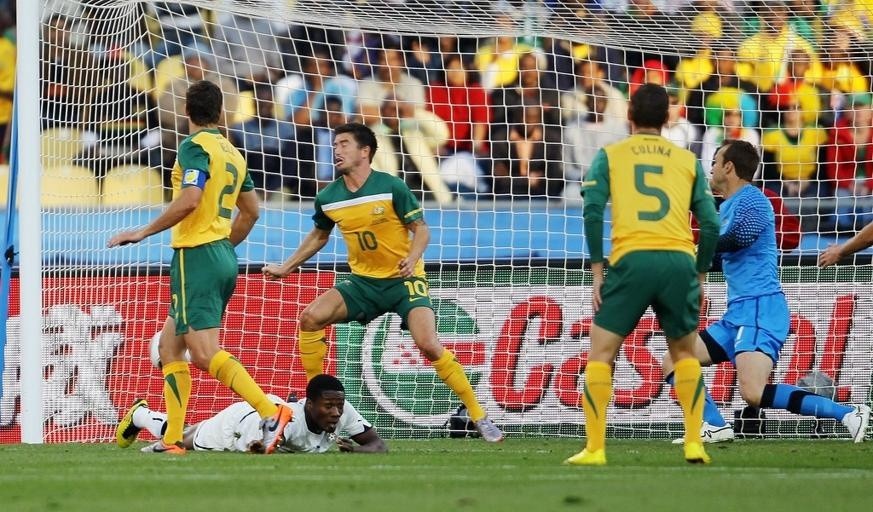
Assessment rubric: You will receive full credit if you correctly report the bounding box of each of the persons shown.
[106,80,293,455]
[818,221,873,270]
[566,84,721,464]
[691,147,800,272]
[0,0,873,225]
[670,140,870,444]
[261,124,504,442]
[116,374,386,454]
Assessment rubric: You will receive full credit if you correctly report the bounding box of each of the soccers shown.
[149,328,194,370]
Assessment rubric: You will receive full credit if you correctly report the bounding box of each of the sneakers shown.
[841,403,872,444]
[684,444,710,464]
[116,398,148,448]
[671,422,734,445]
[139,440,187,454]
[260,402,294,453]
[567,449,606,465]
[472,413,503,443]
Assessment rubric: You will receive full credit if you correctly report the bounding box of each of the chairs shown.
[0,125,164,209]
